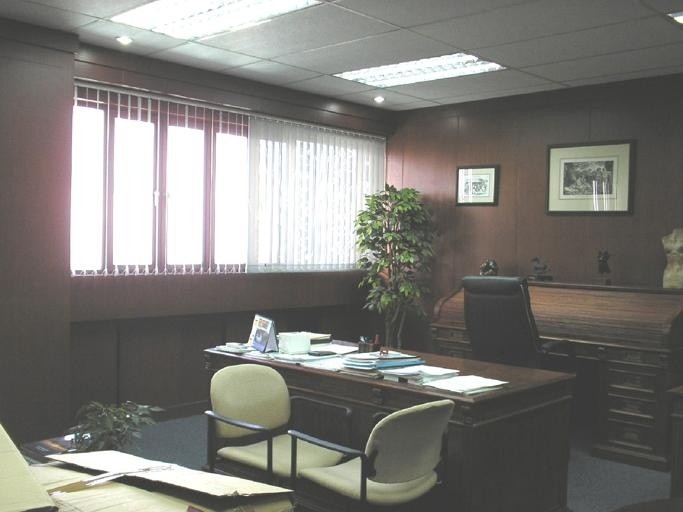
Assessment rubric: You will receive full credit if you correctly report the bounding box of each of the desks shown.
[203,330,576,512]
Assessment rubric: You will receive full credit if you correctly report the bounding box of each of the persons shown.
[660,227,682,288]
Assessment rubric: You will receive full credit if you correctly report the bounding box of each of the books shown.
[343,346,510,398]
[215,330,361,373]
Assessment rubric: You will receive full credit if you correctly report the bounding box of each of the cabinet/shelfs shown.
[428,281,681,474]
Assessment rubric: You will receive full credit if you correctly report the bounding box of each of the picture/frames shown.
[544,139,633,214]
[454,164,498,206]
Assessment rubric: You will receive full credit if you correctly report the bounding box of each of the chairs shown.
[201,364,454,512]
[461,274,542,369]
